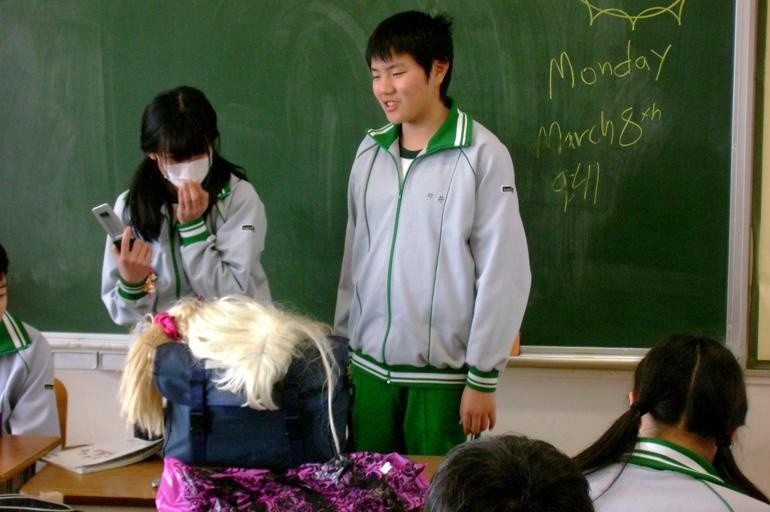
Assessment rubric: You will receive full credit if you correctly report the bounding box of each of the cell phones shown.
[92,203,135,254]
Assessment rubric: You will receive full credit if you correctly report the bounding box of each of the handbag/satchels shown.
[159,336,352,468]
[156,451,428,511]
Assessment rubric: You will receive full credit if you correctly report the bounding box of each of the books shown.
[38,434,166,476]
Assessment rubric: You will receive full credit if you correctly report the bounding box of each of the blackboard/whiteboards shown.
[0,0,760,371]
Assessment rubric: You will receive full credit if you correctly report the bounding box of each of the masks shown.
[153,145,213,189]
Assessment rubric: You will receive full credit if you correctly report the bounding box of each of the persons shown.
[421,432,595,512]
[570,333,769,512]
[333,8,532,454]
[100,86,275,327]
[0,247,67,476]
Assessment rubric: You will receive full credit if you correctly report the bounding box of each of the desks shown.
[20,445,446,511]
[0,433,64,494]
